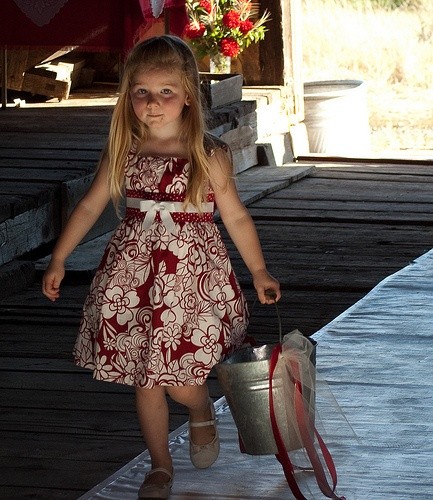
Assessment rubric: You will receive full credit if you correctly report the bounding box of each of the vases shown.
[209,56,231,73]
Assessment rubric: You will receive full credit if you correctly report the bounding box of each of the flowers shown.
[184,0,273,70]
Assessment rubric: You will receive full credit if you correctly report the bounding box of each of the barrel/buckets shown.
[215,292,318,456]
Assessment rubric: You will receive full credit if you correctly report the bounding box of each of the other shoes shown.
[138,465,175,498]
[188,397,219,470]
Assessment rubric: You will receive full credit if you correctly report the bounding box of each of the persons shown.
[43,34,282,499]
